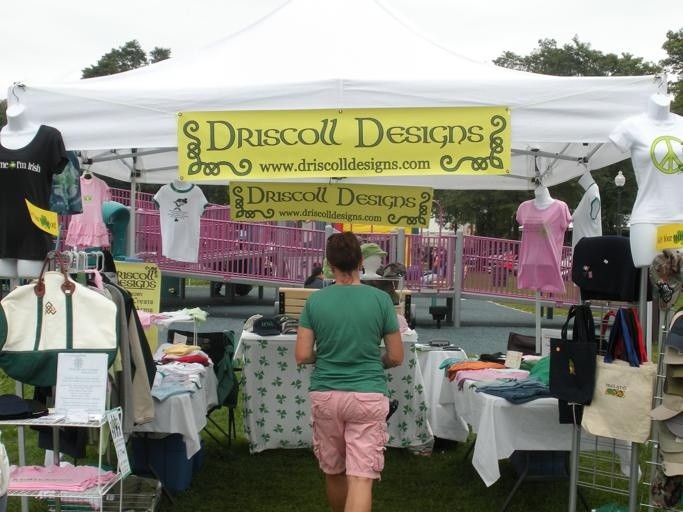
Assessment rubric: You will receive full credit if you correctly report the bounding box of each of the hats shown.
[648,250,683,508]
[359,242,388,260]
[244,314,281,335]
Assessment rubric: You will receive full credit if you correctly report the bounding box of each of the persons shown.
[571,172,602,260]
[0,104,68,280]
[609,96,683,266]
[295,232,404,512]
[493,247,512,288]
[427,244,447,276]
[516,188,564,267]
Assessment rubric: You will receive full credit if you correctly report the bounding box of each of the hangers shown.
[75,160,109,193]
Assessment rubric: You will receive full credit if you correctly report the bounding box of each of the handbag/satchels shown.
[0,250,120,387]
[548,304,658,443]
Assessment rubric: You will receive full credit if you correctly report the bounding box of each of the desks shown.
[234,316,423,458]
[0,413,133,512]
[440,351,592,512]
[133,342,221,505]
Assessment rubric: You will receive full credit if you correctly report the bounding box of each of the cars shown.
[486,252,519,275]
